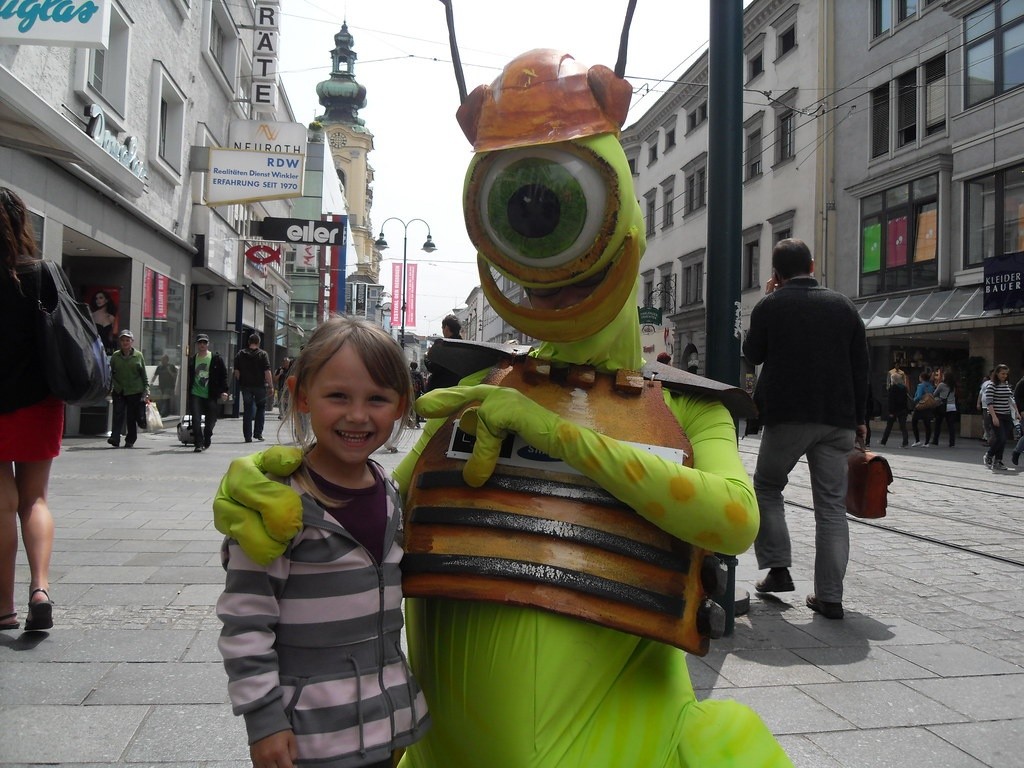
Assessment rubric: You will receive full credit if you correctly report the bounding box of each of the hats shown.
[196,334,210,343]
[120,329,134,338]
[657,353,671,364]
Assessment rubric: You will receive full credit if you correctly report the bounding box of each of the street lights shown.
[373,217,439,347]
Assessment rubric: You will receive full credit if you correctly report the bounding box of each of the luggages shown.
[177,414,206,445]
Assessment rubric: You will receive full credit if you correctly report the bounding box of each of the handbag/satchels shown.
[134,401,147,429]
[916,393,936,409]
[146,402,164,432]
[38,259,114,405]
[932,397,948,413]
[846,436,894,519]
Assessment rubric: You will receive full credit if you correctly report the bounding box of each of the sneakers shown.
[756,569,796,593]
[806,594,846,620]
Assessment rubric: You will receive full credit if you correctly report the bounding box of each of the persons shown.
[213,47,798,768]
[107,328,149,448]
[976,363,1024,470]
[187,333,229,454]
[149,354,179,417]
[384,361,427,453]
[214,314,433,768]
[0,184,77,632]
[233,333,274,443]
[423,315,465,391]
[274,357,291,421]
[742,236,869,620]
[865,361,957,449]
[656,352,672,365]
[89,290,119,355]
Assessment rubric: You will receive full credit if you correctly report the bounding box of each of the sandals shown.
[0,588,54,630]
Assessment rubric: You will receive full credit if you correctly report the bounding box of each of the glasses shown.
[198,341,207,344]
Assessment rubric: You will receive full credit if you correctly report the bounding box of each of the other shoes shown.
[107,437,133,448]
[984,454,1008,470]
[877,440,886,447]
[391,446,399,453]
[911,441,930,448]
[194,442,211,452]
[899,445,909,448]
[245,435,265,443]
